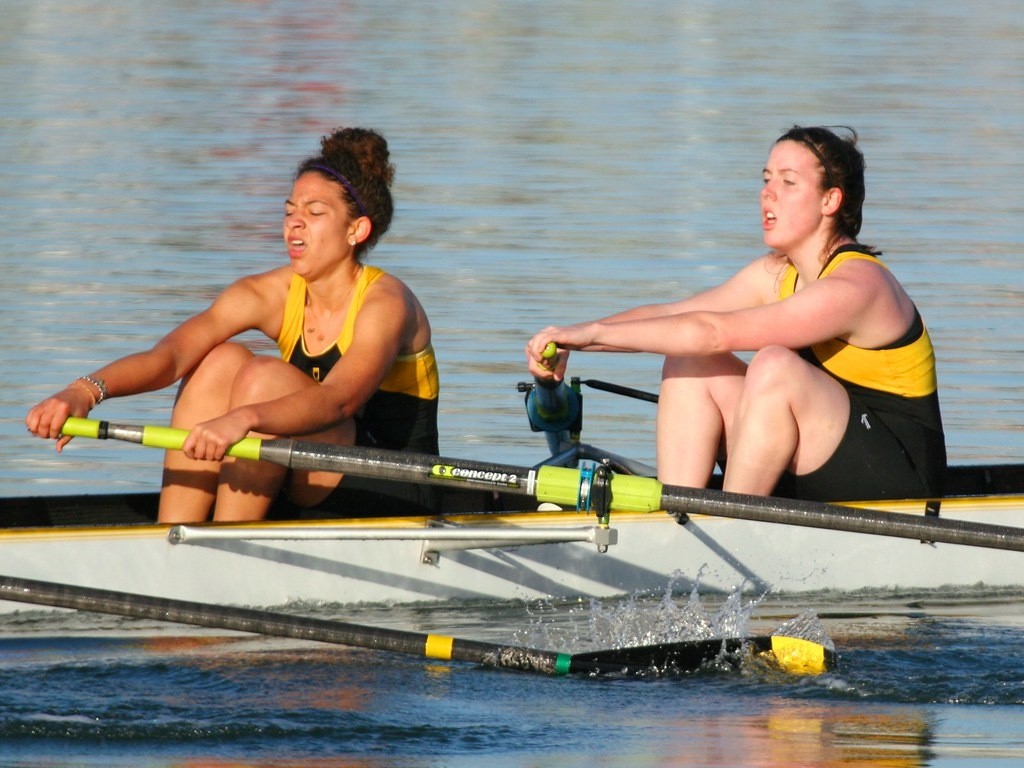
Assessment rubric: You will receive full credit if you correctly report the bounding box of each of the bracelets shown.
[72,374,106,409]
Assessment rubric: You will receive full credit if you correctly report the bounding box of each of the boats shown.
[0,461,1024,617]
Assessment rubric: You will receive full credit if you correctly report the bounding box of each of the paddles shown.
[1,573,843,689]
[57,413,1024,554]
[517,340,586,468]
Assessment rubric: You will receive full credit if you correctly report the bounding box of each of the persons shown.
[526,123,949,510]
[19,127,451,530]
